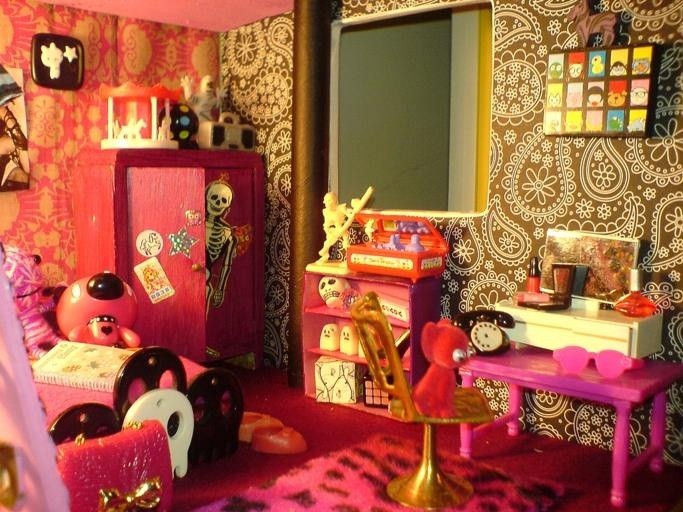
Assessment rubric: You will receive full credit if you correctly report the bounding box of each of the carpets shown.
[195,433,568,511]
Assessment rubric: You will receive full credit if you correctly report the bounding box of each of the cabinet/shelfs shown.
[73,149,264,374]
[301,269,441,424]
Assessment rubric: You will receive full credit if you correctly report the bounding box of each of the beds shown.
[29,345,246,480]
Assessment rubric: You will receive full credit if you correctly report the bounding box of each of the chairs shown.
[350,292,495,512]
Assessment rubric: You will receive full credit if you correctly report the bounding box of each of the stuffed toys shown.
[0,241,60,359]
[403,315,470,418]
